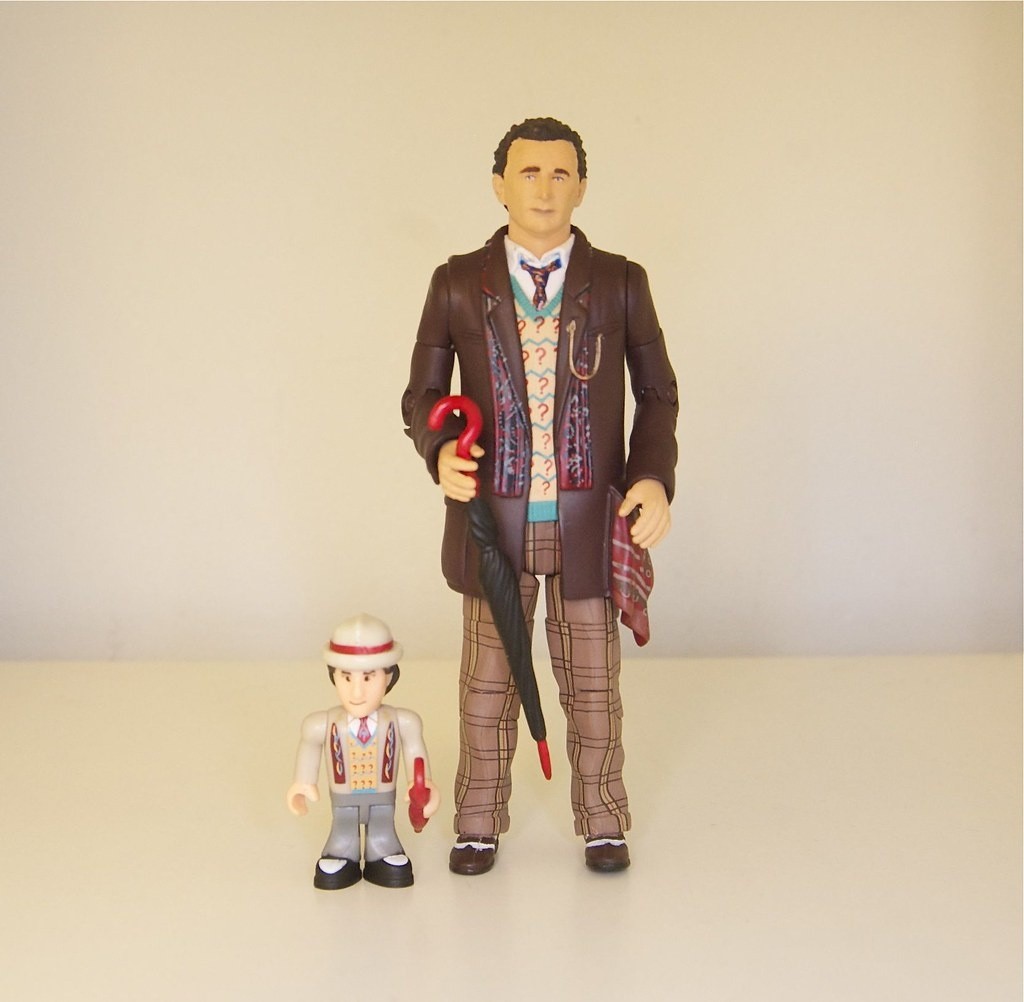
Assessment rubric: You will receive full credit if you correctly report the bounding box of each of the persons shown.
[288,609,441,890]
[401,113,680,872]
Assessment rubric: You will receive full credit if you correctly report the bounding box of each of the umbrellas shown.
[427,396,551,780]
[408,758,431,833]
